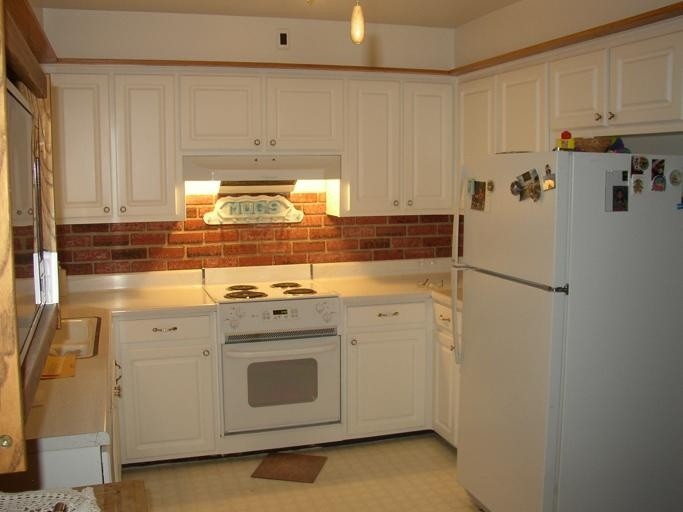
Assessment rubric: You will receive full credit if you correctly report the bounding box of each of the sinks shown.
[46,317,101,358]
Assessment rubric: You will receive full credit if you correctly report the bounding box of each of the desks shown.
[0,480,151,512]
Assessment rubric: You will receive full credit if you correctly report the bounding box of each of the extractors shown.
[180,153,342,182]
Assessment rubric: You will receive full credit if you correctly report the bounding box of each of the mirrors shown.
[6,82,42,367]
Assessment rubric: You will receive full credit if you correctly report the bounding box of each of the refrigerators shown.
[449,153,683,512]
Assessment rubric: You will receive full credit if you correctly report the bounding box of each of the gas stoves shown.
[201,280,336,303]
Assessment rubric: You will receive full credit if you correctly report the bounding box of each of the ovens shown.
[222,336,340,434]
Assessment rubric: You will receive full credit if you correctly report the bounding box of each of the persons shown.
[614,188,626,211]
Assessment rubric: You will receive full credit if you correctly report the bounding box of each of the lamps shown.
[350,1,364,45]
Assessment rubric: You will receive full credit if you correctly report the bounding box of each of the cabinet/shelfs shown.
[325,77,460,217]
[38,362,123,487]
[182,71,345,180]
[434,304,460,443]
[558,18,683,134]
[460,64,546,156]
[48,68,186,223]
[112,311,215,470]
[343,300,435,446]
